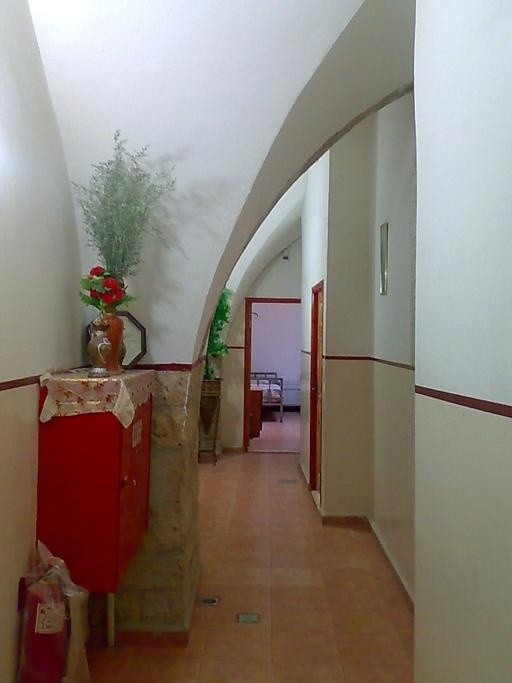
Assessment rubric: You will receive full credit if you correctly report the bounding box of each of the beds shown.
[250,371,283,423]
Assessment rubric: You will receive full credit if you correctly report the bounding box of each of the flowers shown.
[79,268,132,308]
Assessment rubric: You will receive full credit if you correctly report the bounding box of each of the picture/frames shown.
[85,310,147,370]
[379,221,389,295]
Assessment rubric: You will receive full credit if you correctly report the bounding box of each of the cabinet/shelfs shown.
[249,386,263,440]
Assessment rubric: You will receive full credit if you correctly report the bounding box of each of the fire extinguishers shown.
[15,566,70,683]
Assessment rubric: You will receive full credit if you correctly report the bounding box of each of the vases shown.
[103,313,125,373]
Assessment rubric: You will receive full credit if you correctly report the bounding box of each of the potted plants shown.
[200,286,234,436]
